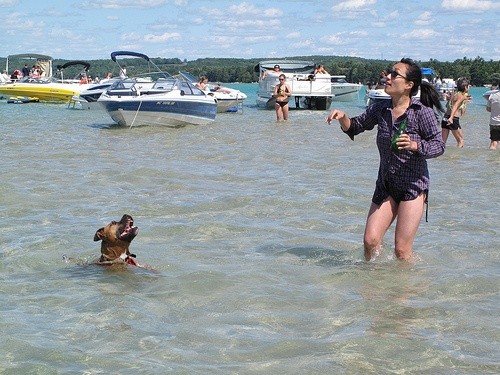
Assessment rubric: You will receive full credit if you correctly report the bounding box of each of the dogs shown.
[87,214,160,275]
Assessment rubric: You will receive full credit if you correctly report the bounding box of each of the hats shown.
[457,78,470,87]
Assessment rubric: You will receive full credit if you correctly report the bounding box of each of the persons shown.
[0,63,129,85]
[486,81,500,149]
[367,69,459,101]
[195,76,209,91]
[270,64,327,74]
[441,78,470,147]
[274,74,292,122]
[326,58,447,263]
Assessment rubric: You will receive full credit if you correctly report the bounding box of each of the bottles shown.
[389,117,408,154]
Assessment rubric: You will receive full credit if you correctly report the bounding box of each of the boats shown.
[158,71,248,113]
[367,67,498,105]
[0,53,99,104]
[253,58,335,110]
[77,75,159,110]
[330,74,368,103]
[99,50,219,129]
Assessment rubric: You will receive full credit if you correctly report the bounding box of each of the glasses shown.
[279,78,284,81]
[386,68,412,82]
